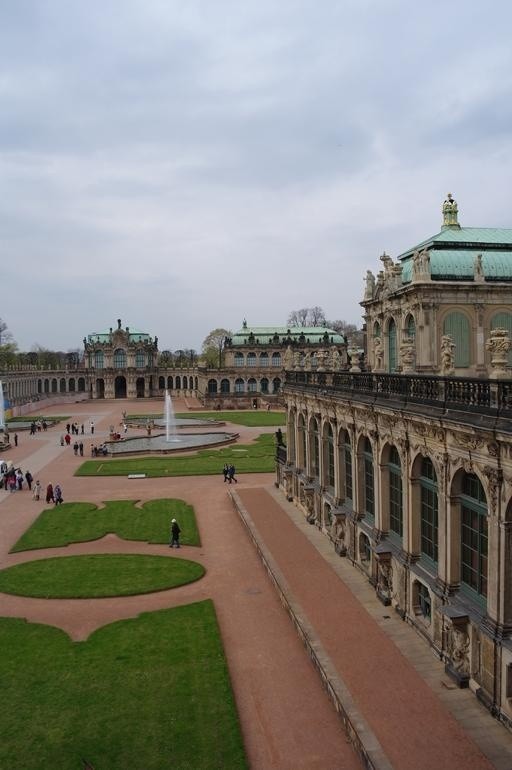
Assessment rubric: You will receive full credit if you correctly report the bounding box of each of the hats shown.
[171,519,176,522]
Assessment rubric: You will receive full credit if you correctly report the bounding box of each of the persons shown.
[3,464,63,506]
[168,518,181,548]
[223,463,238,483]
[29,419,48,435]
[146,423,152,435]
[14,433,18,446]
[124,423,127,433]
[59,422,121,457]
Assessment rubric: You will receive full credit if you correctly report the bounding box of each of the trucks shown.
[0,460,20,488]
